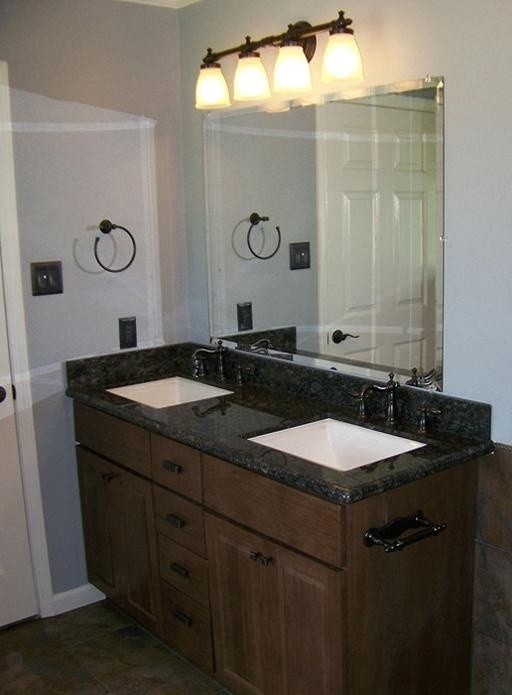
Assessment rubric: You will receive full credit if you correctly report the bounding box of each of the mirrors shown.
[202,76,445,390]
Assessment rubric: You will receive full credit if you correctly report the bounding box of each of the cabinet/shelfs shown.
[74,398,480,695]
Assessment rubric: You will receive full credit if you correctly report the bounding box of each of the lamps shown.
[195,11,367,110]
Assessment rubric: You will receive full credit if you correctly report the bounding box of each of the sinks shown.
[234,409,429,475]
[104,375,235,410]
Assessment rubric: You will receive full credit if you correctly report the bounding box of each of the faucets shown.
[406,365,439,388]
[357,372,403,426]
[189,396,232,417]
[235,334,273,356]
[190,339,229,383]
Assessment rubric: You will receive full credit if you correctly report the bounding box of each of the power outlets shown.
[118,316,137,349]
[236,303,254,332]
